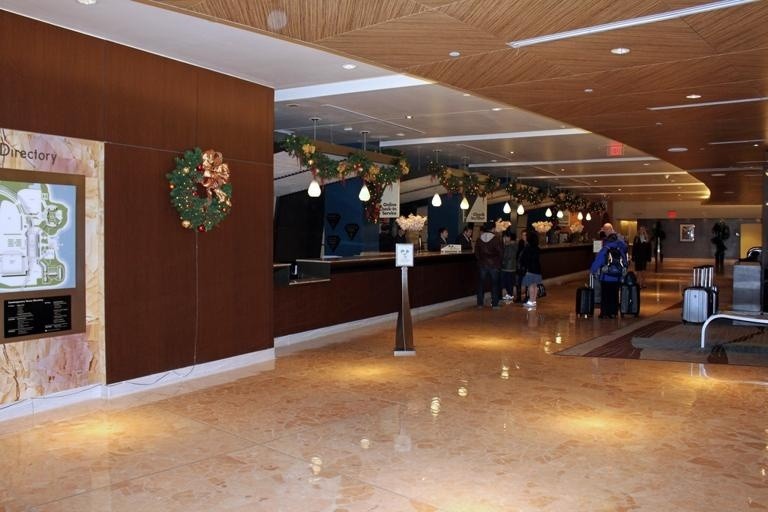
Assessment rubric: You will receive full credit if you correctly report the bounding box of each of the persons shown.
[378,219,653,319]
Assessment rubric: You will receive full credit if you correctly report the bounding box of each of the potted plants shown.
[710,216,731,273]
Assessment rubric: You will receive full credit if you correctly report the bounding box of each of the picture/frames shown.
[679,223,695,243]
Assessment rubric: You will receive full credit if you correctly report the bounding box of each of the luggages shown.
[577,273,639,318]
[682,265,719,325]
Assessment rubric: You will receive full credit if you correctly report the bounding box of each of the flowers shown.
[569,221,585,233]
[394,212,429,233]
[530,219,554,233]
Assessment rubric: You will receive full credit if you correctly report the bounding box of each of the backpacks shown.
[607,246,624,273]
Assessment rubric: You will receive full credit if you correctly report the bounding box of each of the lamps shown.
[307,114,322,199]
[358,130,372,204]
[430,148,592,222]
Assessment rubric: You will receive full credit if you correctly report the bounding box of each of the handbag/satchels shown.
[526,284,545,298]
[626,271,636,286]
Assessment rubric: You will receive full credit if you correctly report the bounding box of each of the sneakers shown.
[502,295,514,300]
[523,301,536,307]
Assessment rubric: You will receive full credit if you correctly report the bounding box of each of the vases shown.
[404,230,419,254]
[538,231,547,248]
